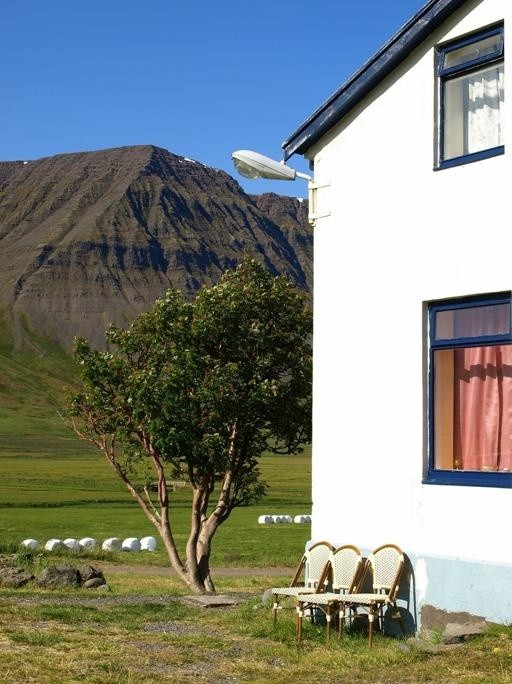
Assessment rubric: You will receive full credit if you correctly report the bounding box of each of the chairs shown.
[271,542,407,649]
